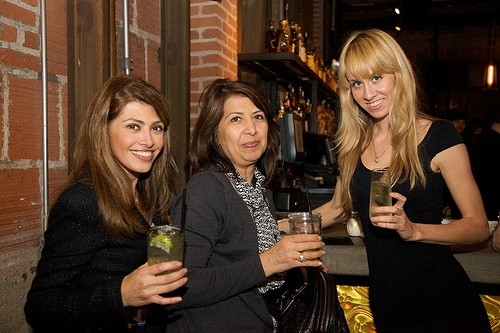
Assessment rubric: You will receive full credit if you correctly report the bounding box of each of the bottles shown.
[268,18,340,96]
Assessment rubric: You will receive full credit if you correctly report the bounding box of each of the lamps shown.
[479,0,500,90]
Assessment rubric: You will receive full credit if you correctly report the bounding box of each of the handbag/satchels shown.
[269,269,351,333]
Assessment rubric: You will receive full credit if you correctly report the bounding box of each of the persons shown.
[426,107,500,221]
[279,30,492,333]
[24,75,188,333]
[163,78,326,333]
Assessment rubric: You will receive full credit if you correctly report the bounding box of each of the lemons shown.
[149,235,173,254]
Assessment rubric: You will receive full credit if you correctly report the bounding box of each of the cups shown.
[146,226,183,276]
[369,168,393,226]
[288,211,323,263]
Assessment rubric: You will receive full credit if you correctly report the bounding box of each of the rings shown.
[297,252,306,262]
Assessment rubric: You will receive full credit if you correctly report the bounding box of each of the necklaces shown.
[134,191,139,204]
[371,137,391,163]
